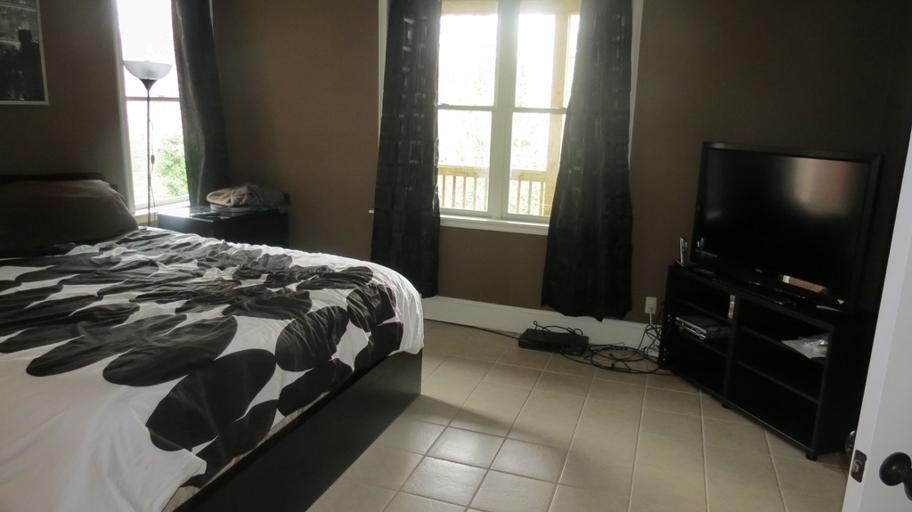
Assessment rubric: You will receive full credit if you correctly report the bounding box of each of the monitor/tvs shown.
[689,139,889,320]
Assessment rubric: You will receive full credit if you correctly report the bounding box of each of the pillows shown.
[0,180,137,257]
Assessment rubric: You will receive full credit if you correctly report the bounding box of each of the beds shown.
[0,171,424,512]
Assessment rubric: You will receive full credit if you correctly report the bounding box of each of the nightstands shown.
[158,206,288,247]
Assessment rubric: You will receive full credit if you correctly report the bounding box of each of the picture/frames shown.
[0,0,51,106]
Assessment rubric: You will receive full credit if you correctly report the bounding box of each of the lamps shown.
[122,60,173,226]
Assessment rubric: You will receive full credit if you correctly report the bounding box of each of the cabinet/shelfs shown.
[657,263,876,460]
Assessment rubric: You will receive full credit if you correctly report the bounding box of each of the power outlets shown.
[644,296,657,315]
